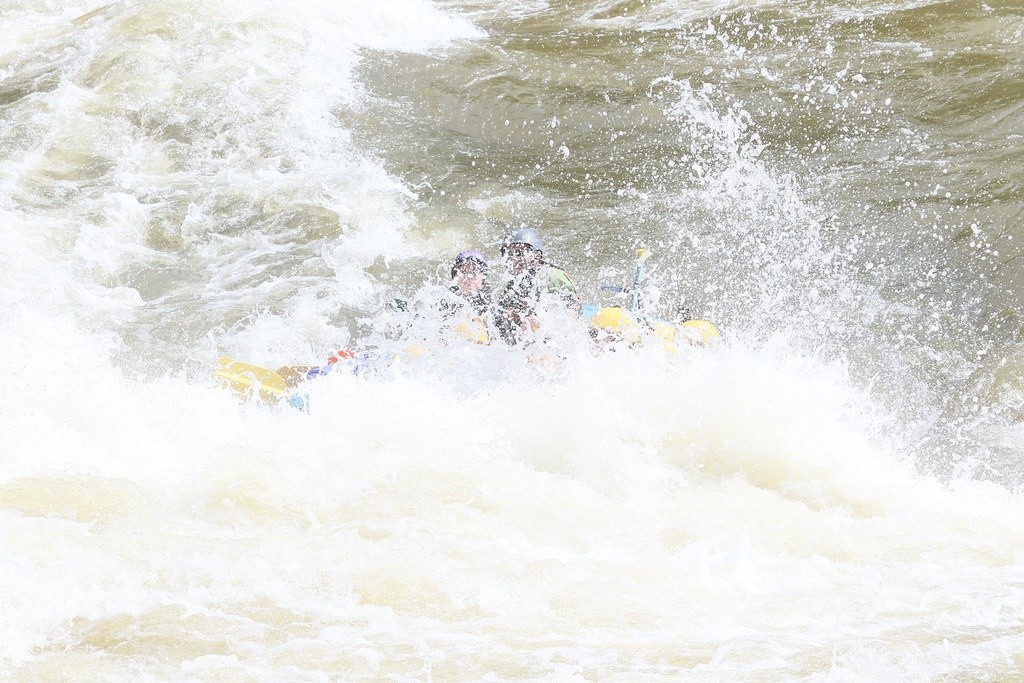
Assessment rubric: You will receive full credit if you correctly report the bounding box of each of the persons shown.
[492,228,583,348]
[446,252,515,345]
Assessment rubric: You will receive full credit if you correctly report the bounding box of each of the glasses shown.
[503,249,528,257]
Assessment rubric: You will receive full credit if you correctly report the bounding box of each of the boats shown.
[211,304,723,409]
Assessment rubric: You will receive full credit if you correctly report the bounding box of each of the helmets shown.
[451,250,488,279]
[500,227,543,258]
